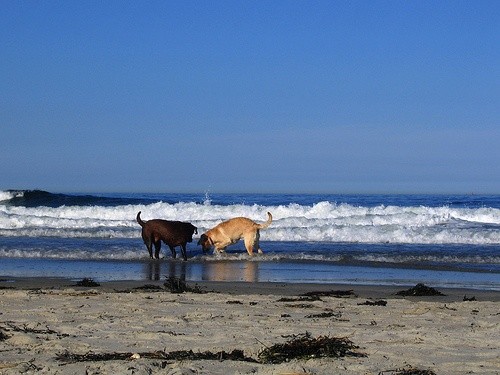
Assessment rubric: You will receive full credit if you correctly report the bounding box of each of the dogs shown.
[136,211,197,261]
[197,212,272,256]
